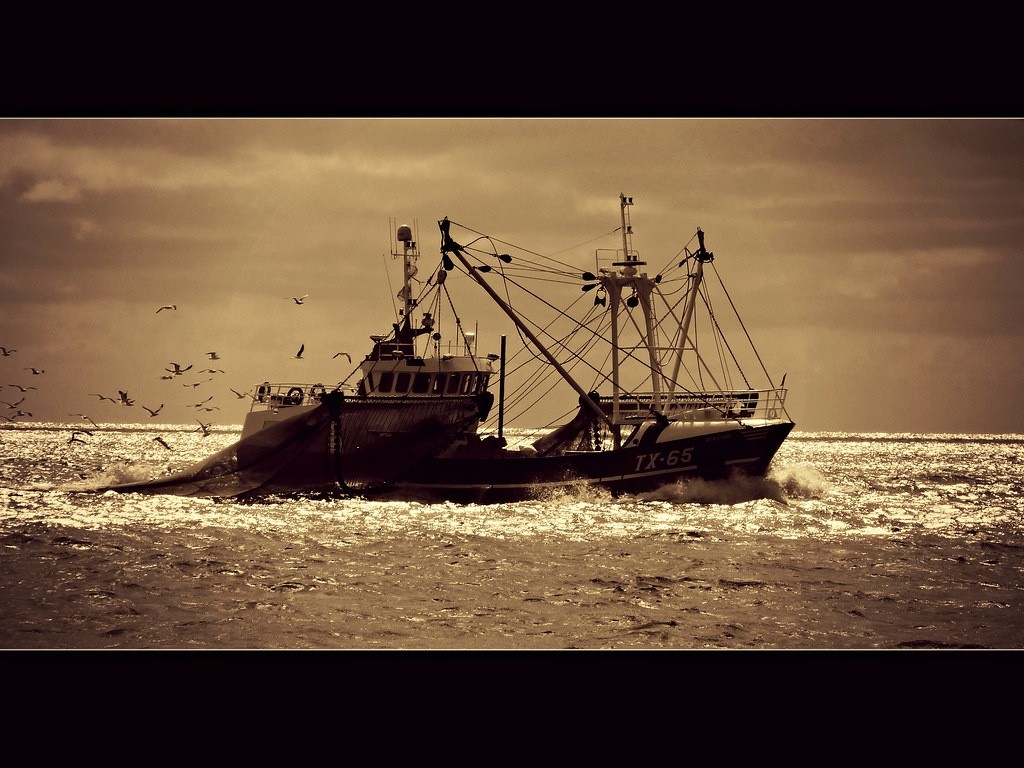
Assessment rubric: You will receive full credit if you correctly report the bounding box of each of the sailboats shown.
[405,192,797,500]
[69,216,495,503]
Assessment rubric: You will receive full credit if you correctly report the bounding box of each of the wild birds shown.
[155,303,177,314]
[332,352,352,364]
[290,344,306,361]
[230,388,247,399]
[284,294,311,306]
[0,344,226,451]
